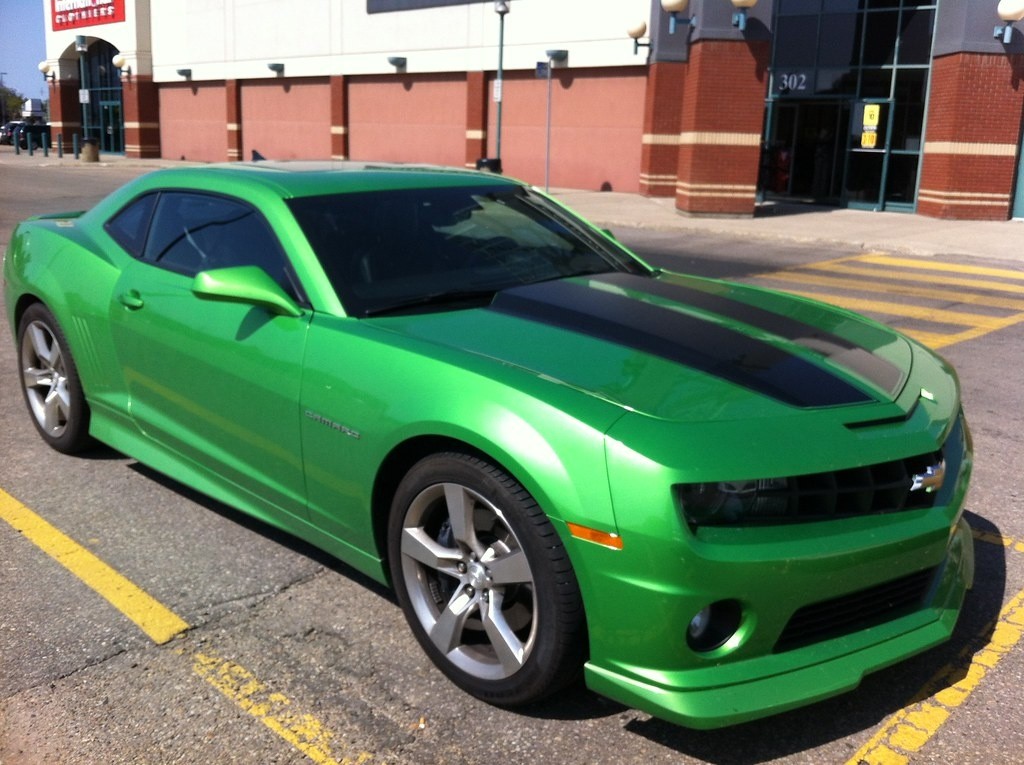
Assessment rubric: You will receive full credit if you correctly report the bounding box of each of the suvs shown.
[12,124,50,150]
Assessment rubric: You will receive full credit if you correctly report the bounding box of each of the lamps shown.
[994,0,1024,43]
[660,0,696,35]
[388,56,407,68]
[39,61,56,81]
[268,63,284,70]
[177,69,190,75]
[112,55,132,77]
[623,21,653,54]
[728,0,757,29]
[547,51,570,62]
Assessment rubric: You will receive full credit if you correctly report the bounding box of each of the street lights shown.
[74,34,101,163]
[495,3,512,170]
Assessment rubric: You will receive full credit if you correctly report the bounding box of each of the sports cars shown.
[11,160,984,739]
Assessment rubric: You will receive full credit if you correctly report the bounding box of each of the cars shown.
[0,121,19,144]
